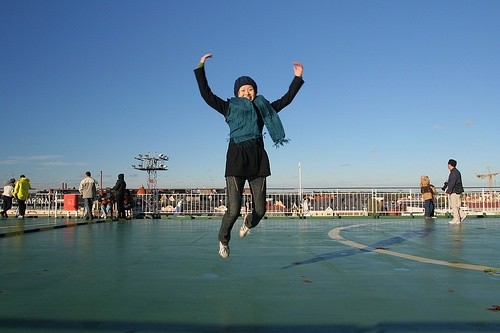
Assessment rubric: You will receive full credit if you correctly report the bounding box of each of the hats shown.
[234,76,258,97]
[449,159,457,164]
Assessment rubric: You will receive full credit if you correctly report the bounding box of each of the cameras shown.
[441,182,449,191]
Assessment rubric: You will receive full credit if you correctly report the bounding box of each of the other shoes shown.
[0,211,4,218]
[17,215,24,219]
[5,214,8,218]
[117,216,126,219]
[219,241,230,258]
[461,215,467,222]
[449,221,460,224]
[240,215,250,238]
[104,214,107,219]
[424,216,434,219]
[101,214,104,217]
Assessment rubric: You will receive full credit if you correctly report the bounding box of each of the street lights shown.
[131,152,169,218]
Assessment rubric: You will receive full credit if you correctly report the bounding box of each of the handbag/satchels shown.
[93,182,96,199]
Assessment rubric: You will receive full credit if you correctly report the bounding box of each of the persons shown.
[98,187,113,219]
[79,172,96,220]
[112,174,126,218]
[421,176,437,219]
[446,159,467,224]
[13,175,30,218]
[0,178,15,218]
[194,54,304,257]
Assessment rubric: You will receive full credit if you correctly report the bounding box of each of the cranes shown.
[474,166,500,194]
